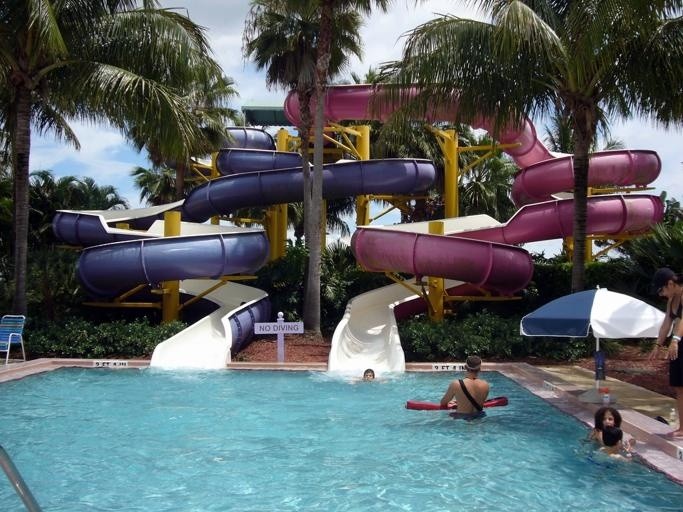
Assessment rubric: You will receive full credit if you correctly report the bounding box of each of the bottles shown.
[669,408,677,427]
[603,394,610,403]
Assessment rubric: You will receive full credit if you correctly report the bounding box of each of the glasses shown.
[655,283,666,295]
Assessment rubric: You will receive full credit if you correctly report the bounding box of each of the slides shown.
[52,85,663,372]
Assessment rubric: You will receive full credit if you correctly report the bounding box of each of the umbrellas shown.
[519,288,674,404]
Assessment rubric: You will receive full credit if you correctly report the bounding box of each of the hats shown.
[650,267,674,296]
[464,355,483,370]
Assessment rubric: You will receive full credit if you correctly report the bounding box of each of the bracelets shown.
[672,335,681,341]
[626,453,632,457]
[656,343,662,348]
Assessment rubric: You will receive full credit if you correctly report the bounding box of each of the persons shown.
[440,356,490,420]
[587,406,636,453]
[599,426,633,463]
[364,368,374,379]
[646,266,683,440]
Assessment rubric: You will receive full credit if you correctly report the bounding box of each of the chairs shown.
[0,314,27,364]
